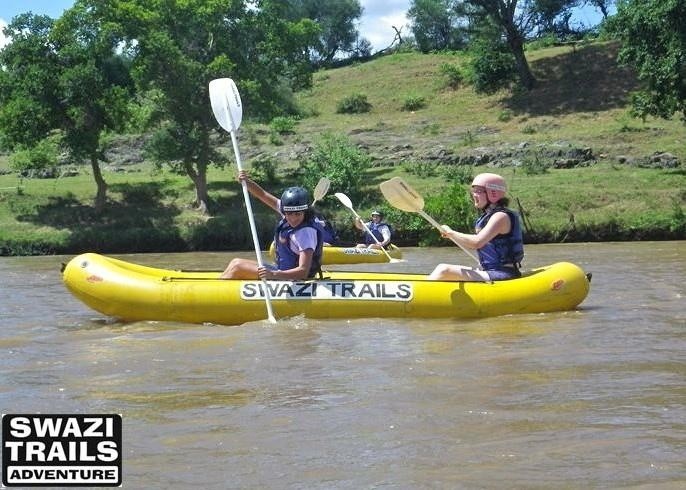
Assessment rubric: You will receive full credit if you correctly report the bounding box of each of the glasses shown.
[473,189,486,194]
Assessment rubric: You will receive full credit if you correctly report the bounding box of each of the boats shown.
[60,241,593,322]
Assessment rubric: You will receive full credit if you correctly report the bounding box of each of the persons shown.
[426,172,524,282]
[354,207,393,251]
[220,169,326,281]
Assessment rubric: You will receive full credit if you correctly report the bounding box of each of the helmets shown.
[471,172,506,203]
[277,186,309,215]
[371,210,381,217]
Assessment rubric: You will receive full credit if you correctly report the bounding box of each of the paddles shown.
[311,177,331,206]
[208,78,277,325]
[381,176,482,269]
[334,193,401,263]
